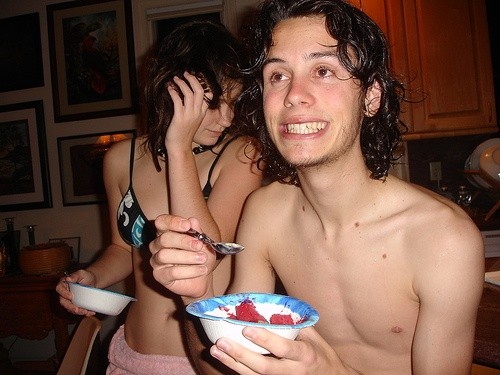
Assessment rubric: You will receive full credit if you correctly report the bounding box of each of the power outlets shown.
[430,161,442,182]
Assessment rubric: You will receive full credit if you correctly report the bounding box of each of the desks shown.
[0,262,89,375]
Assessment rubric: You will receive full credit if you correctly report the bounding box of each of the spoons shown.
[169,228,245,255]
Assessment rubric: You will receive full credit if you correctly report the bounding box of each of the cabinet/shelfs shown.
[344,0,498,140]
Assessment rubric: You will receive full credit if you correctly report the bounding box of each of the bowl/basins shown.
[463,138,500,189]
[19,243,66,276]
[68,279,134,316]
[185,292,320,354]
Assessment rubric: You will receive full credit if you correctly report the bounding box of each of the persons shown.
[55,20,267,375]
[147,2,487,375]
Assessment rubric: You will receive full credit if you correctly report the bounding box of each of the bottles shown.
[428,144,442,191]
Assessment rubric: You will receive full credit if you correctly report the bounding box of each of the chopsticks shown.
[62,271,80,284]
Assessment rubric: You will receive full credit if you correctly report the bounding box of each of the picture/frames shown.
[0,99,53,212]
[46,0,140,123]
[56,129,138,206]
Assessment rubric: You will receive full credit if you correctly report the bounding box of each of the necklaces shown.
[156,132,226,158]
